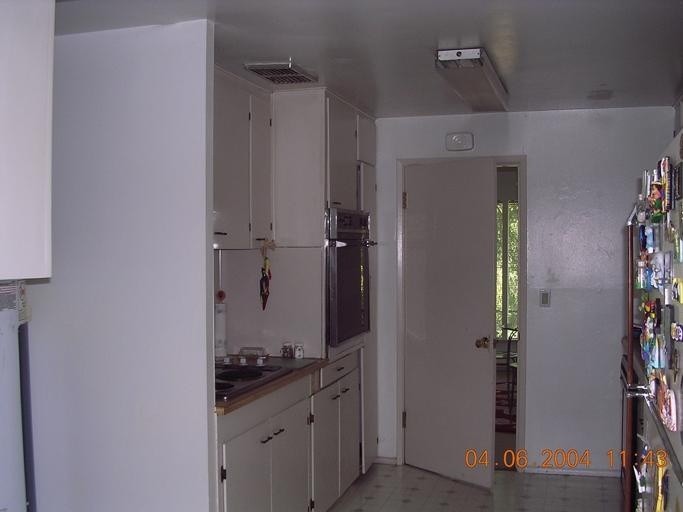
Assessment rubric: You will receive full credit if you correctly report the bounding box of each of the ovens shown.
[325,208,378,349]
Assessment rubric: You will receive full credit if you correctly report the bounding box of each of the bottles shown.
[294,343,305,360]
[282,343,293,359]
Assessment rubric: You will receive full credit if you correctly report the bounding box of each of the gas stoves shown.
[212,365,294,403]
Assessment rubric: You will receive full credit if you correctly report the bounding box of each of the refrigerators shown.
[620,205,647,510]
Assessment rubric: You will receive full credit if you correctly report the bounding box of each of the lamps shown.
[432,46,510,114]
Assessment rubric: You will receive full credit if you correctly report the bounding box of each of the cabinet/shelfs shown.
[214,64,272,249]
[273,87,377,247]
[312,342,377,512]
[218,398,310,511]
